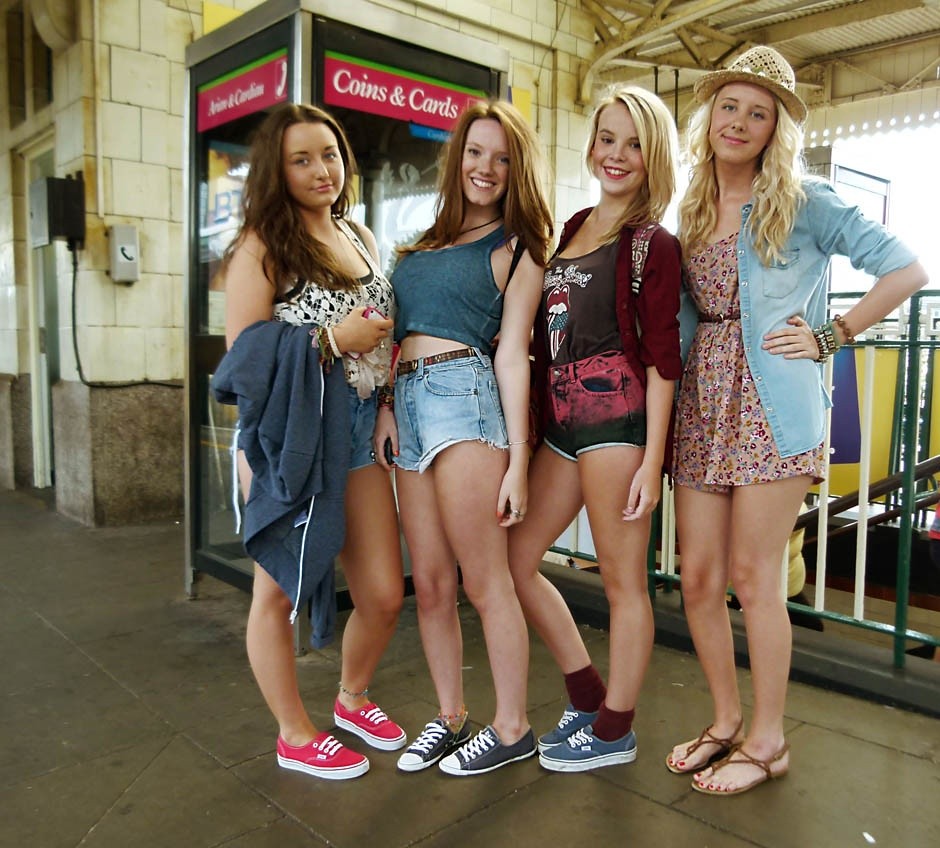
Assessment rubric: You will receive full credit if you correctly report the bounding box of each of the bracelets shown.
[309,324,344,374]
[812,321,841,363]
[378,394,393,407]
[508,440,530,445]
[835,315,855,347]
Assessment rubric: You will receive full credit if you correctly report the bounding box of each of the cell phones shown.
[345,306,389,360]
[386,438,392,465]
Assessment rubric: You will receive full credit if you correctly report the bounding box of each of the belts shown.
[398,347,485,376]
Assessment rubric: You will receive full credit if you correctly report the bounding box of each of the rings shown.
[518,512,525,519]
[511,510,519,518]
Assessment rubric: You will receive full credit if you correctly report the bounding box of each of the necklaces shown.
[457,215,502,234]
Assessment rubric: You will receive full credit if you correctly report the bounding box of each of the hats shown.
[693,44,807,125]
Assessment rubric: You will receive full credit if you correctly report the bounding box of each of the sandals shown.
[691,739,792,795]
[665,715,744,774]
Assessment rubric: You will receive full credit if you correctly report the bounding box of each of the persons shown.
[374,100,554,776]
[215,103,408,782]
[508,85,684,773]
[666,45,930,796]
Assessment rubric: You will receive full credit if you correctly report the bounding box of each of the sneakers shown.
[540,723,638,773]
[441,726,537,777]
[537,705,602,753]
[276,734,371,781]
[396,713,472,772]
[333,692,407,751]
